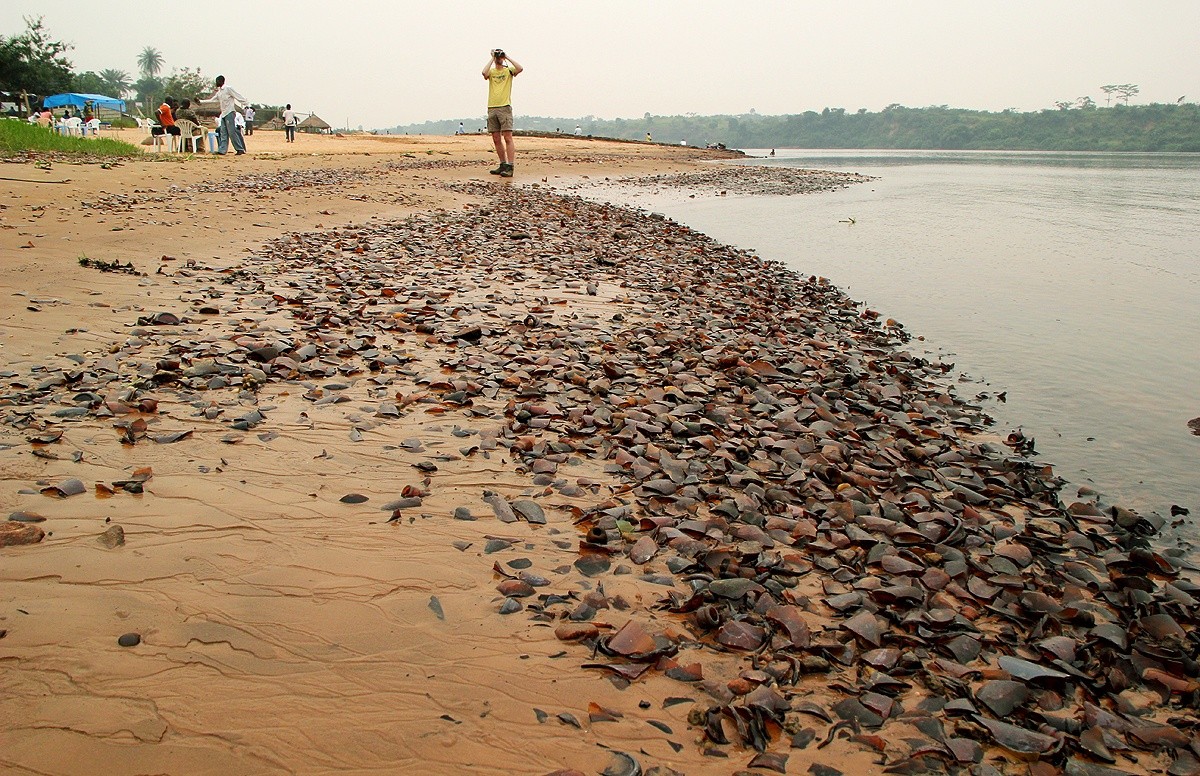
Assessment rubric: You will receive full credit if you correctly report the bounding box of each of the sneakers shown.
[500,165,513,177]
[490,162,507,175]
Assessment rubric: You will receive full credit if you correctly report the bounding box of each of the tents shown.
[45,92,127,129]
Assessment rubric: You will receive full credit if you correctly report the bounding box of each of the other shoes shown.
[213,152,224,155]
[235,150,245,155]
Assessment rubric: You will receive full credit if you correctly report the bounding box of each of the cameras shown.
[495,52,506,57]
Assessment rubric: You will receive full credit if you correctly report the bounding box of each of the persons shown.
[482,48,523,176]
[456,120,487,134]
[769,148,775,155]
[282,104,296,143]
[273,120,276,128]
[8,107,16,116]
[373,129,422,136]
[154,75,256,155]
[33,101,101,126]
[556,125,582,136]
[680,139,686,146]
[646,133,652,142]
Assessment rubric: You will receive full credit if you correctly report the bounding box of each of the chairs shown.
[146,117,220,156]
[28,112,101,136]
